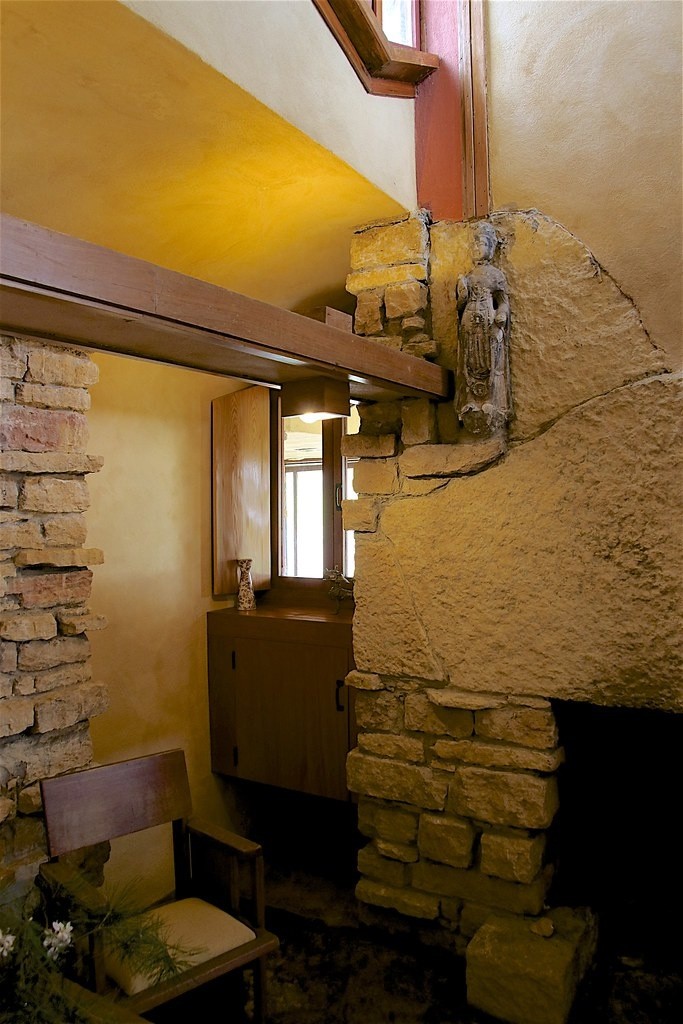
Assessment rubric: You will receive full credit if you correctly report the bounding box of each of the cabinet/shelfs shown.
[205,611,353,801]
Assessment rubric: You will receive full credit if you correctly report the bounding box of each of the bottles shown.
[235,558,257,611]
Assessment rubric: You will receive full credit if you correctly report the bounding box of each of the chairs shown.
[0,869,153,1024]
[34,747,280,1024]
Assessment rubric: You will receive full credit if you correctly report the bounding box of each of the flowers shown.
[0,863,203,1024]
